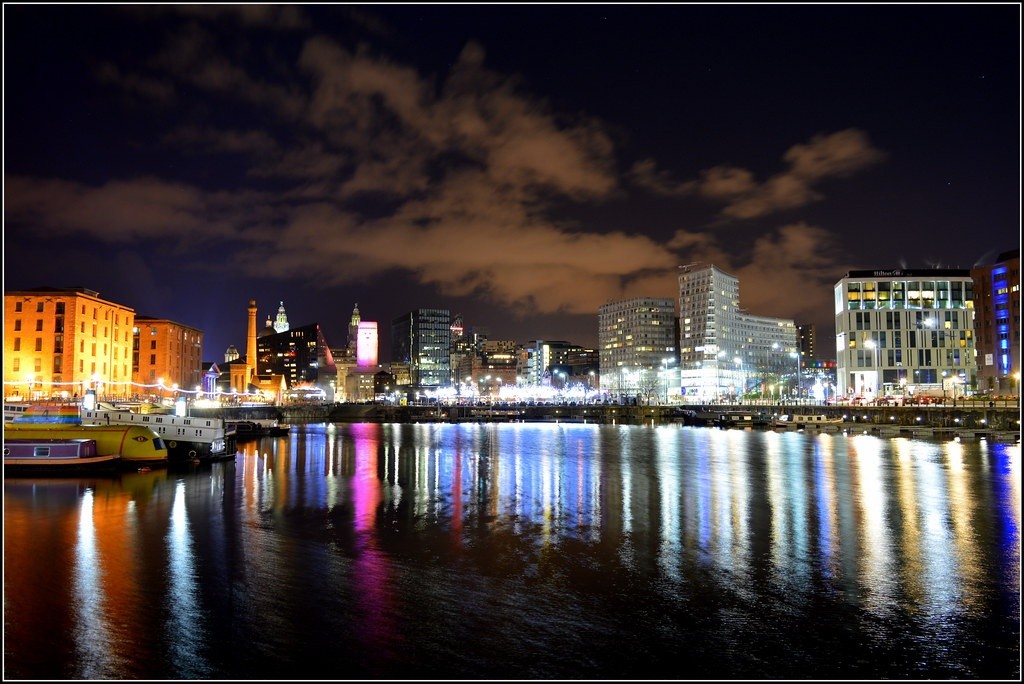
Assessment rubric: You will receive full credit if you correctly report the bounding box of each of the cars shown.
[873,394,941,406]
[851,397,868,405]
[822,400,834,406]
[840,398,850,402]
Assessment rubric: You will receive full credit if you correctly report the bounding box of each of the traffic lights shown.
[802,362,839,368]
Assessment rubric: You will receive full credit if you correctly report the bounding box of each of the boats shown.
[4,437,121,468]
[767,413,844,430]
[467,407,526,422]
[78,412,226,463]
[708,410,770,427]
[410,410,448,422]
[224,418,290,438]
[3,421,169,462]
[659,408,696,417]
[224,422,264,441]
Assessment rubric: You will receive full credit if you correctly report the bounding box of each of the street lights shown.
[622,366,629,395]
[714,349,726,404]
[915,318,934,392]
[660,358,670,405]
[734,355,746,404]
[449,367,598,390]
[765,342,780,403]
[790,350,804,399]
[90,372,260,406]
